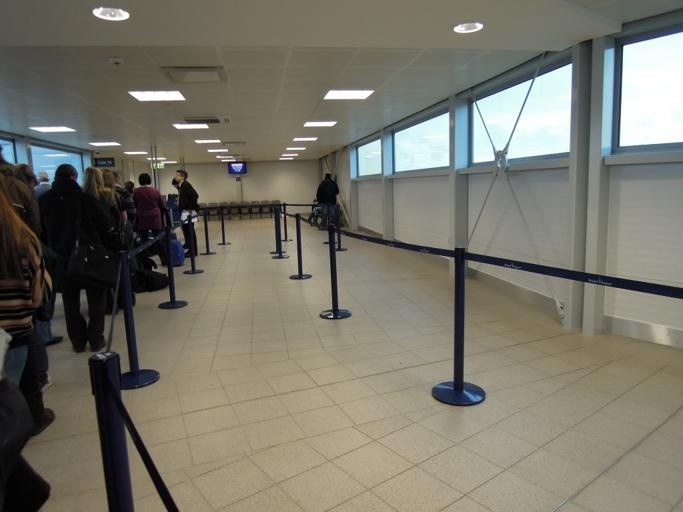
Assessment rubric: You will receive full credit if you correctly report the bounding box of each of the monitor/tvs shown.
[227,162,247,174]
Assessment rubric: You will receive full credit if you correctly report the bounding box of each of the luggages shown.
[163,208,185,266]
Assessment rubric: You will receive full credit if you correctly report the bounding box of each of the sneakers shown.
[183,244,197,257]
[45,335,106,353]
[41,375,53,394]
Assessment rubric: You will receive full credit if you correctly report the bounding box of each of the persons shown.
[307,198,322,227]
[316,170,339,230]
[0,147,198,512]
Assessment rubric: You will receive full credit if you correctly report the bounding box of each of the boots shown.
[25,392,55,436]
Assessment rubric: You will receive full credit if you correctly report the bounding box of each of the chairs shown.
[198,200,282,221]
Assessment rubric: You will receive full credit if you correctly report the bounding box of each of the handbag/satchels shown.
[65,244,116,286]
